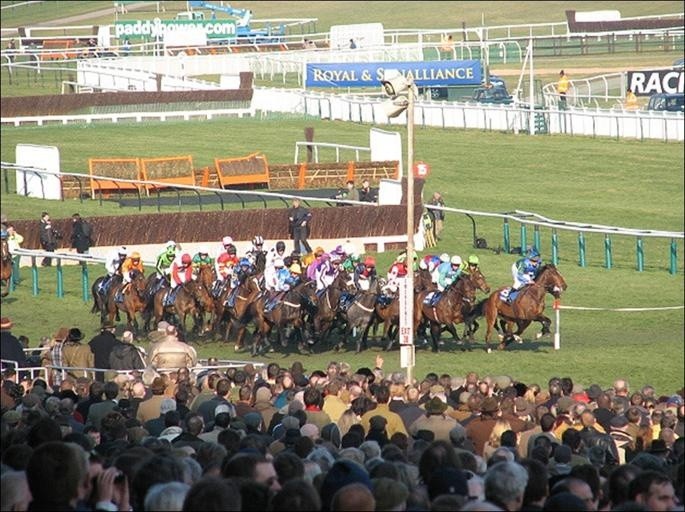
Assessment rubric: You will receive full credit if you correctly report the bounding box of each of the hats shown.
[3,411,21,424]
[514,396,532,417]
[289,362,308,373]
[149,377,169,390]
[424,396,448,414]
[53,327,69,341]
[100,320,117,329]
[1,317,15,330]
[412,430,435,442]
[113,398,135,413]
[67,328,86,341]
[477,397,500,414]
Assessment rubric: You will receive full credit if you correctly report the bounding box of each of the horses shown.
[374,270,423,352]
[384,265,433,351]
[466,261,568,354]
[0,237,12,297]
[299,267,357,354]
[327,275,395,353]
[208,273,263,353]
[494,284,561,345]
[248,276,320,357]
[99,270,148,338]
[417,265,490,339]
[255,250,268,273]
[143,271,170,332]
[141,277,215,343]
[195,263,213,337]
[414,274,476,354]
[91,276,120,321]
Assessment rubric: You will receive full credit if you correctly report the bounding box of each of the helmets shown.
[364,256,375,267]
[330,254,342,264]
[227,246,237,255]
[252,235,264,245]
[199,246,209,254]
[468,255,479,265]
[291,251,301,260]
[451,255,461,265]
[118,247,129,256]
[222,236,234,247]
[182,254,191,264]
[440,252,450,262]
[314,248,324,257]
[166,241,177,249]
[131,252,141,260]
[276,242,285,251]
[528,250,540,261]
[351,253,361,262]
[240,258,250,267]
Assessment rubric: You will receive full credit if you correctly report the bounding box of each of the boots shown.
[428,290,441,307]
[505,287,519,305]
[98,274,111,295]
[149,278,160,295]
[212,279,222,295]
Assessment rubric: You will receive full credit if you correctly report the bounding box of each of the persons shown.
[441,34,454,52]
[39,212,61,267]
[99,236,545,314]
[347,37,356,49]
[0,214,25,287]
[87,39,97,58]
[623,88,640,110]
[1,316,685,511]
[5,39,16,63]
[210,7,218,20]
[557,69,568,108]
[288,198,314,254]
[422,191,447,248]
[329,179,380,208]
[301,38,317,50]
[70,213,95,265]
[28,39,38,66]
[122,36,130,57]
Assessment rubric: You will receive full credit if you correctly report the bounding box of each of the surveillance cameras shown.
[380,75,410,100]
